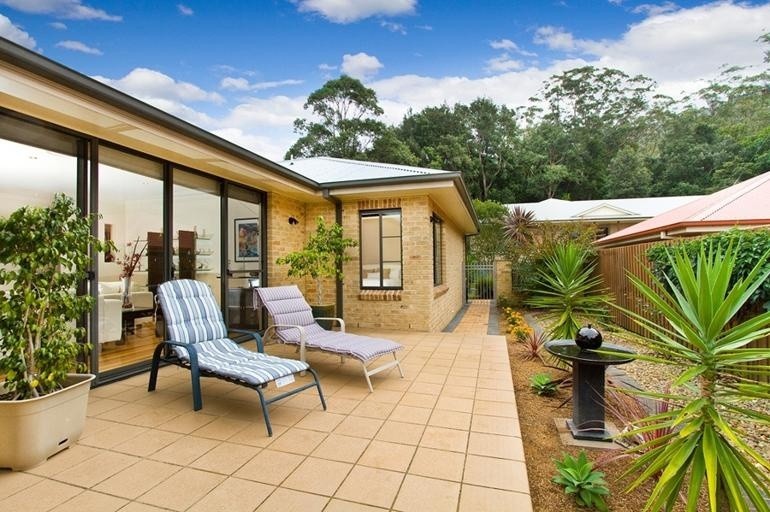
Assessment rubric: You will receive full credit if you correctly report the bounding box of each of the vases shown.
[121,276,133,308]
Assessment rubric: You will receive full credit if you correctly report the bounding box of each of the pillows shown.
[96,281,122,295]
[362,268,391,281]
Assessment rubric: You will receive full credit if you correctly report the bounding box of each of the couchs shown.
[49,280,154,352]
[362,264,401,288]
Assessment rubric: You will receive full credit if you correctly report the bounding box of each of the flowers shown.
[117,237,148,307]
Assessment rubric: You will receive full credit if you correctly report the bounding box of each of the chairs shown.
[148,281,327,436]
[252,284,405,394]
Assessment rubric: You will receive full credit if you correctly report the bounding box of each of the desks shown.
[543,337,639,442]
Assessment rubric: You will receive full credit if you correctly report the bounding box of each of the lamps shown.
[289,217,299,225]
[430,215,441,223]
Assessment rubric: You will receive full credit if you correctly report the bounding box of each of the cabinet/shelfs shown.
[134,237,215,272]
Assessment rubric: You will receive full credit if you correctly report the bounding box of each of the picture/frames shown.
[234,216,259,262]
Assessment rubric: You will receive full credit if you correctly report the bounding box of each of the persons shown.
[239,224,257,257]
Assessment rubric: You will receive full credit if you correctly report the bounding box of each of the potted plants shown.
[277,213,361,330]
[0,190,117,472]
